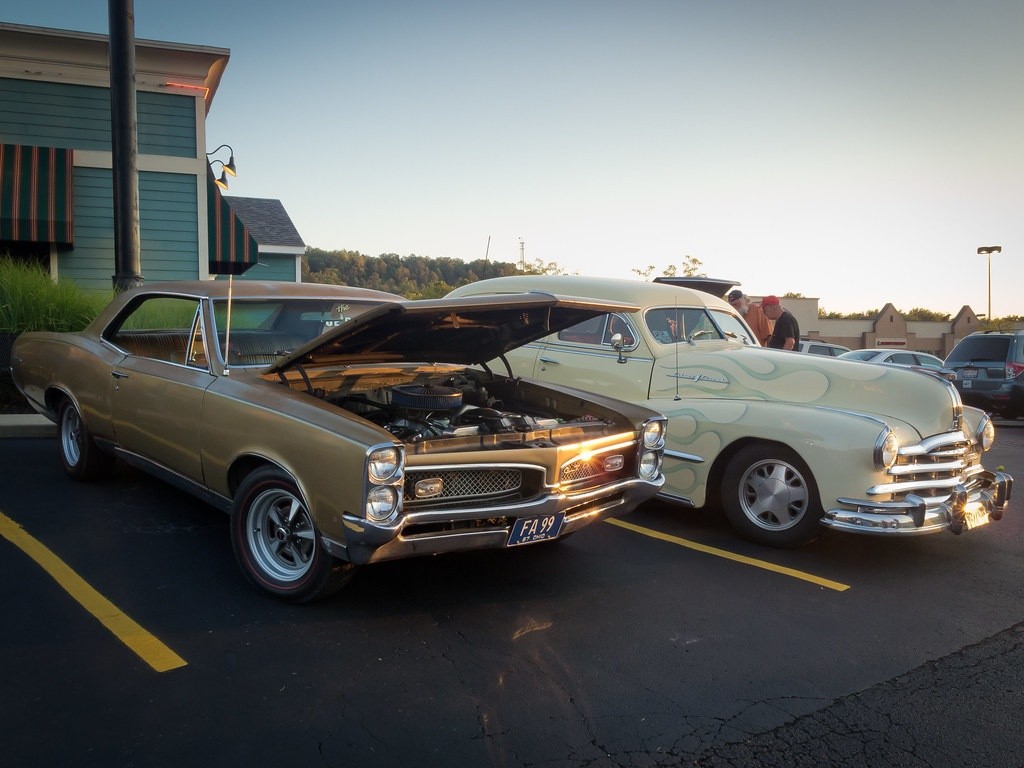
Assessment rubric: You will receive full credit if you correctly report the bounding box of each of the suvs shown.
[799,337,862,361]
[942,329,1024,419]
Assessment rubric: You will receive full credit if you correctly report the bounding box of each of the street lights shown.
[977,246,1002,326]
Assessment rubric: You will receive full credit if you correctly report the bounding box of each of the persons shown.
[757,295,800,352]
[728,290,774,347]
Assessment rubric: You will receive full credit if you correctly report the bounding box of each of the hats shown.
[728,290,742,303]
[758,295,779,307]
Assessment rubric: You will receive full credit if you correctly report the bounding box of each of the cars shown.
[836,349,943,375]
[9,275,667,602]
[441,276,1014,541]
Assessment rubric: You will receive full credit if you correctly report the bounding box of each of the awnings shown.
[0,143,75,252]
[207,159,258,276]
